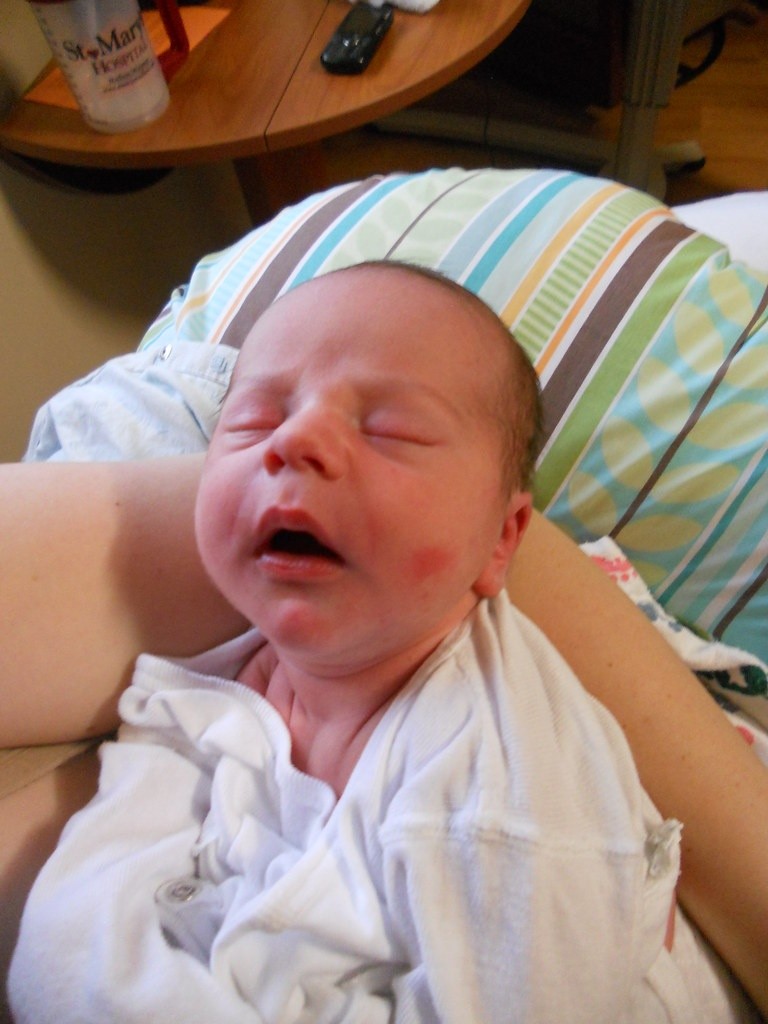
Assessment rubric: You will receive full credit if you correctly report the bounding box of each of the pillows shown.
[135,166,768,668]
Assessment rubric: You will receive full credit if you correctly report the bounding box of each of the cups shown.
[26,0,191,136]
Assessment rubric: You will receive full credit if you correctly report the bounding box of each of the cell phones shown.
[321,3,396,75]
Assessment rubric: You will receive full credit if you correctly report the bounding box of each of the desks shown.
[0,0,531,227]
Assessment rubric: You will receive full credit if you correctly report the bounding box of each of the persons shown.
[0,260,768,1024]
